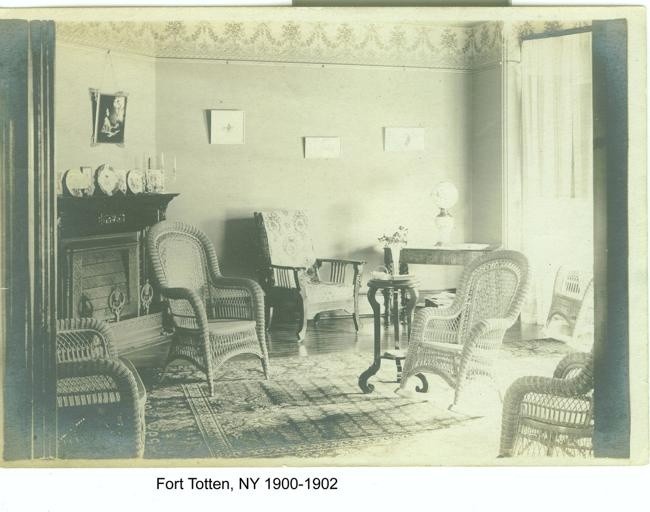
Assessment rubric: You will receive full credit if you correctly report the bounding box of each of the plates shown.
[65,163,144,197]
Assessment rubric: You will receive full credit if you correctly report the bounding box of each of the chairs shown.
[55,317,148,459]
[147,207,367,396]
[393,249,595,457]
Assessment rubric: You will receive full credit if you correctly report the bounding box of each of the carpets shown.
[180,369,465,459]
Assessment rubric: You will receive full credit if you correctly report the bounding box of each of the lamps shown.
[432,182,458,246]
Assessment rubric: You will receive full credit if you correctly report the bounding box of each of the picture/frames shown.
[208,109,246,146]
[90,87,130,147]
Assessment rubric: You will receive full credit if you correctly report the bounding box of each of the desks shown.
[359,278,430,394]
[398,242,494,325]
